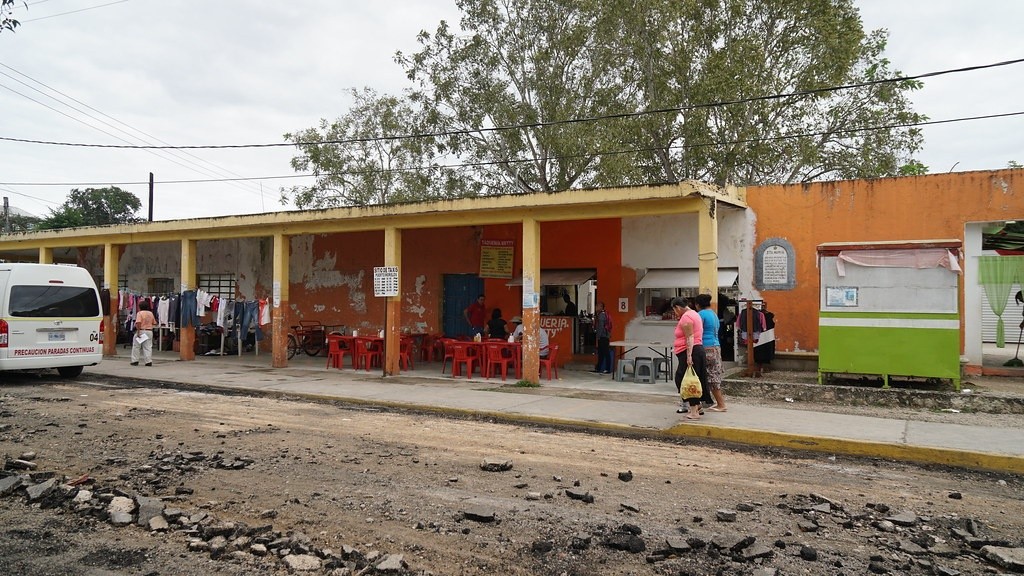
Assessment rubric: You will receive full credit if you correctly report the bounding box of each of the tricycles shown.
[287,319,347,360]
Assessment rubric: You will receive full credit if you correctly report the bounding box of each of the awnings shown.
[505,269,596,285]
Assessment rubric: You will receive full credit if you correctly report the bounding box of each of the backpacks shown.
[602,310,612,333]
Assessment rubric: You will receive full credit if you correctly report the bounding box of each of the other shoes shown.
[145,362,152,366]
[589,370,603,374]
[130,362,138,366]
[676,406,688,413]
[600,371,613,376]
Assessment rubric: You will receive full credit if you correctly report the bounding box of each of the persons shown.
[676,294,728,412]
[513,315,549,356]
[484,309,510,338]
[563,294,576,315]
[463,295,486,335]
[130,301,157,366]
[669,296,706,417]
[589,302,611,376]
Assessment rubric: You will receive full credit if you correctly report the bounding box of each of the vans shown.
[0,262,104,379]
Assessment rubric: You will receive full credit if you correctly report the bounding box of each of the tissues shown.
[508,336,514,343]
[379,330,384,338]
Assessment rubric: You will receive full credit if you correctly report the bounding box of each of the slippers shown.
[706,406,725,412]
[680,415,700,420]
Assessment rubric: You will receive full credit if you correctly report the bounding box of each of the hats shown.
[509,316,522,322]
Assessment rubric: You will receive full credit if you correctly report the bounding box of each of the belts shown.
[140,329,153,331]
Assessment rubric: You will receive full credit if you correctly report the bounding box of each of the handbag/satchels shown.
[680,366,703,399]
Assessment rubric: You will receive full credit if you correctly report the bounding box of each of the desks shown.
[610,342,675,382]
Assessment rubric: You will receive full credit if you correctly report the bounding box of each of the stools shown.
[653,357,672,380]
[617,359,634,381]
[635,360,656,383]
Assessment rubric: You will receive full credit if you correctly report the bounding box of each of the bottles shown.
[357,329,360,336]
[378,328,385,338]
[473,332,481,342]
[507,333,514,343]
[663,310,679,320]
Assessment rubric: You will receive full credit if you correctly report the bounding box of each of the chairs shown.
[324,331,560,381]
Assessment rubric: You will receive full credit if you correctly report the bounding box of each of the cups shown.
[353,330,357,336]
